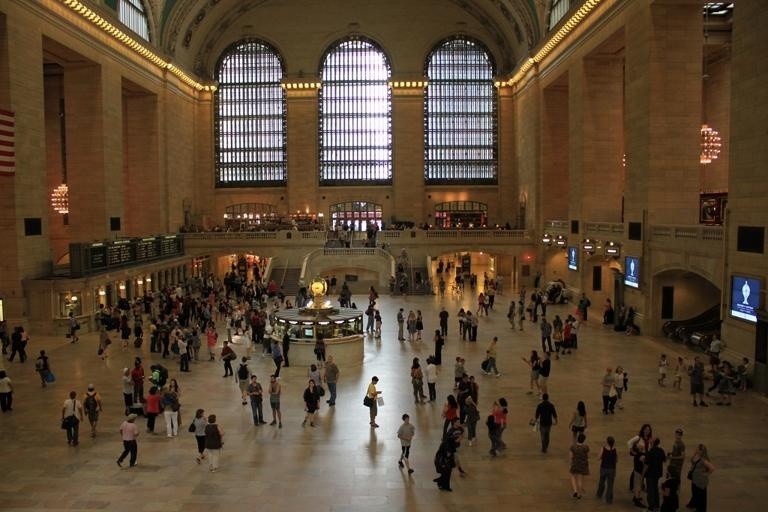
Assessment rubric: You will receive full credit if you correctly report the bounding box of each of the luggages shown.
[134,336,143,348]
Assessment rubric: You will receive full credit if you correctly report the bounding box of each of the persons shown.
[0,218,750,512]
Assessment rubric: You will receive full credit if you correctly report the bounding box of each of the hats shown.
[675,428,683,433]
[87,384,94,389]
[123,367,128,373]
[127,413,138,420]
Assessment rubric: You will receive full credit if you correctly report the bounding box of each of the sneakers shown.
[72,336,80,344]
[398,459,414,475]
[196,457,220,474]
[632,495,647,508]
[116,460,122,467]
[326,399,335,406]
[66,432,99,446]
[693,399,709,408]
[370,420,380,428]
[254,417,282,429]
[223,372,233,378]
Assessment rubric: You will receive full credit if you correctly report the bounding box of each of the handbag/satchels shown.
[687,469,693,480]
[228,351,237,360]
[363,395,385,407]
[61,413,79,429]
[482,359,492,373]
[188,417,195,433]
[47,372,55,383]
[608,384,618,398]
[98,337,113,356]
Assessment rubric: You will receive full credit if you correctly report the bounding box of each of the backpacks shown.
[84,393,99,415]
[35,358,44,371]
[238,363,249,380]
